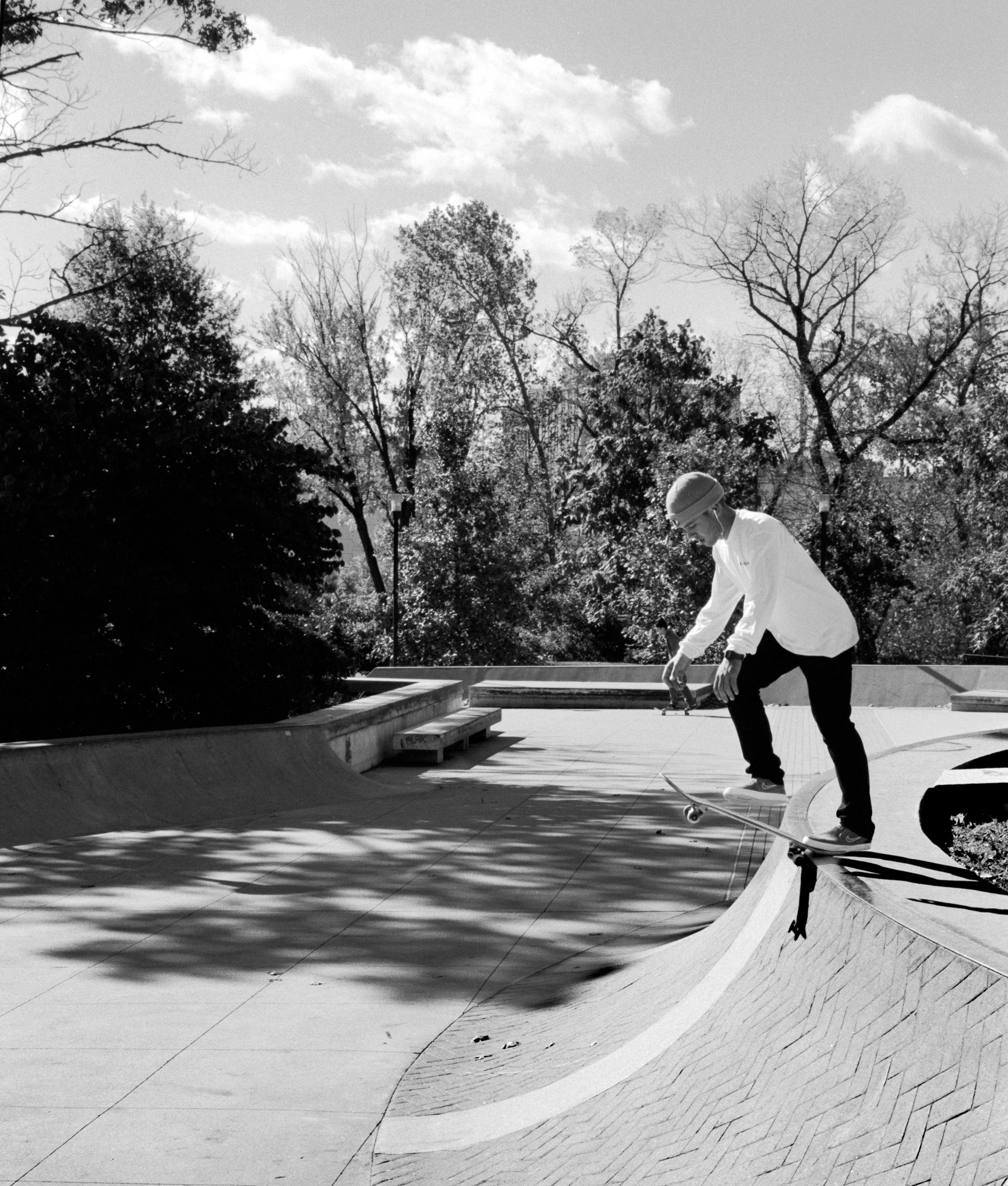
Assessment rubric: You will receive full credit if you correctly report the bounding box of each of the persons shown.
[656,617,696,711]
[662,472,876,851]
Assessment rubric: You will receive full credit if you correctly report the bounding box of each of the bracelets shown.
[724,650,746,661]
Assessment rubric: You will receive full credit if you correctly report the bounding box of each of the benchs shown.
[950,690,1008,712]
[468,680,713,707]
[393,707,502,762]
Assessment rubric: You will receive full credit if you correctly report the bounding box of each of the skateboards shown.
[658,772,845,855]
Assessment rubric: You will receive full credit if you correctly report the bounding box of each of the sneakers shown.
[685,703,697,710]
[801,823,871,852]
[663,704,679,711]
[723,777,787,806]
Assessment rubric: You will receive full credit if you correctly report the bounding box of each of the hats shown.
[666,472,724,528]
[655,617,668,628]
